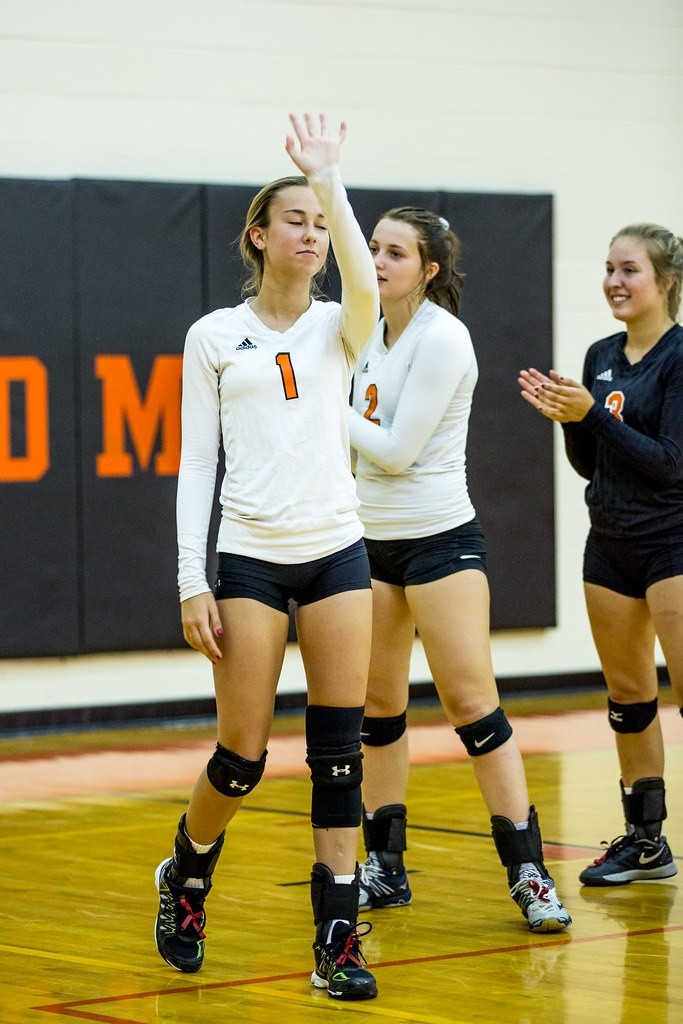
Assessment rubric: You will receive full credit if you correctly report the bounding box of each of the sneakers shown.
[311,921,378,1000]
[509,869,572,933]
[579,833,678,887]
[154,857,212,973]
[358,857,412,912]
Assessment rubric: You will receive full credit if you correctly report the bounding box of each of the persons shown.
[515,222,683,886]
[348,207,573,934]
[156,108,385,1000]
